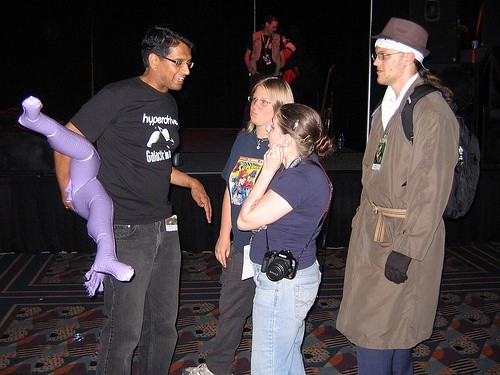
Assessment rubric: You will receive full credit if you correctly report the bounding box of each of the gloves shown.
[384,250,412,284]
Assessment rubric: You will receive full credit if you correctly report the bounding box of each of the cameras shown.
[261,249,297,281]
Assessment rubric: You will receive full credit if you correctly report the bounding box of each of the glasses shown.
[153,51,194,69]
[372,51,403,61]
[247,96,271,106]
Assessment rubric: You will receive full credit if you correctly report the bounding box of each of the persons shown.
[188,76,295,375]
[18,96,134,296]
[52,27,212,375]
[244,15,319,111]
[335,16,460,375]
[236,104,334,375]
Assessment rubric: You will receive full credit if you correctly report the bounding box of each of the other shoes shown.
[182,363,234,375]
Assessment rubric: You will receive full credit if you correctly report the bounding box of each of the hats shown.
[369,17,429,57]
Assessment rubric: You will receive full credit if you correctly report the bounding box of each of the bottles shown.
[338,133,346,150]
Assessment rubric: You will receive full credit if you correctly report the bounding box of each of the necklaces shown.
[253,129,268,150]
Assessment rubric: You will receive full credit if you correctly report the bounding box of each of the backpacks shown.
[401,85,482,221]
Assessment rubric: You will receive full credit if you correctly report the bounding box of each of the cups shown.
[471,38,480,63]
